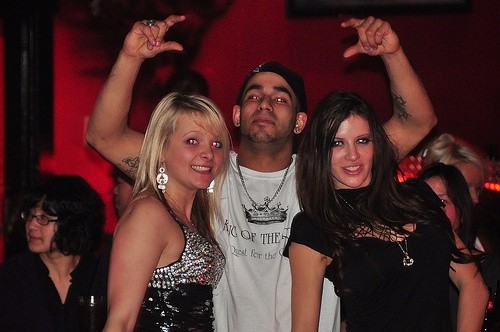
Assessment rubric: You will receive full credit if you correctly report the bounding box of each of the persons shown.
[85,14,440,332]
[102,88,233,332]
[110,164,141,219]
[281,90,489,332]
[420,132,500,254]
[165,67,211,99]
[414,160,499,332]
[0,171,111,332]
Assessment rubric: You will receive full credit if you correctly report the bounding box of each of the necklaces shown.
[235,152,293,213]
[166,191,198,232]
[339,188,415,268]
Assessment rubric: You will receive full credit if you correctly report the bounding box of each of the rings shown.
[146,19,157,28]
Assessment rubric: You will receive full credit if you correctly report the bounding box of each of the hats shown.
[237,61,307,113]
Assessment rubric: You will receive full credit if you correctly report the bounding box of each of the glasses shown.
[21,210,59,225]
